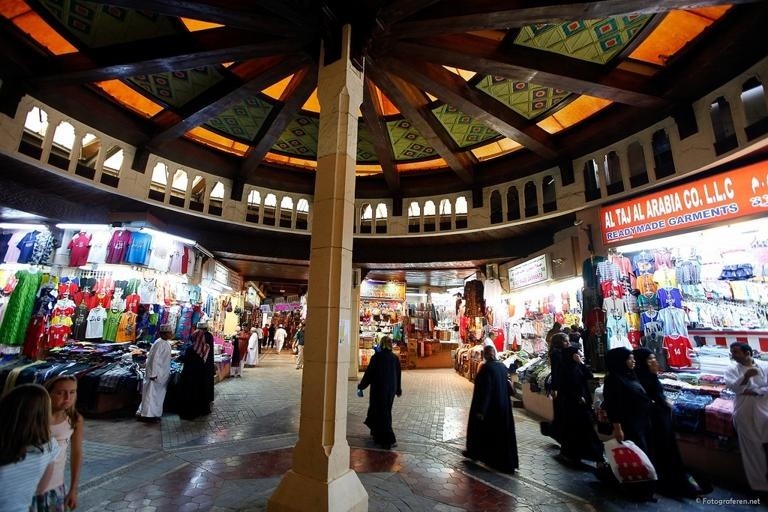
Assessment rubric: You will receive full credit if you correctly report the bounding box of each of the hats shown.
[160,325,171,332]
[197,322,207,328]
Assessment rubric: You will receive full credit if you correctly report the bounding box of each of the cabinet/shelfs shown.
[464,228,768,511]
[0,220,263,416]
[358,246,463,370]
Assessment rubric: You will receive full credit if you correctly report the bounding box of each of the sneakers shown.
[461,449,478,461]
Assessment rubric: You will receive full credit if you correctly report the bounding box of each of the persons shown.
[195,322,215,410]
[356,335,403,451]
[230,324,241,338]
[0,382,63,511]
[0,265,43,347]
[133,322,174,423]
[245,326,260,368]
[229,321,251,378]
[255,323,306,370]
[461,345,521,475]
[724,342,768,506]
[538,321,711,506]
[28,374,85,511]
[172,329,211,422]
